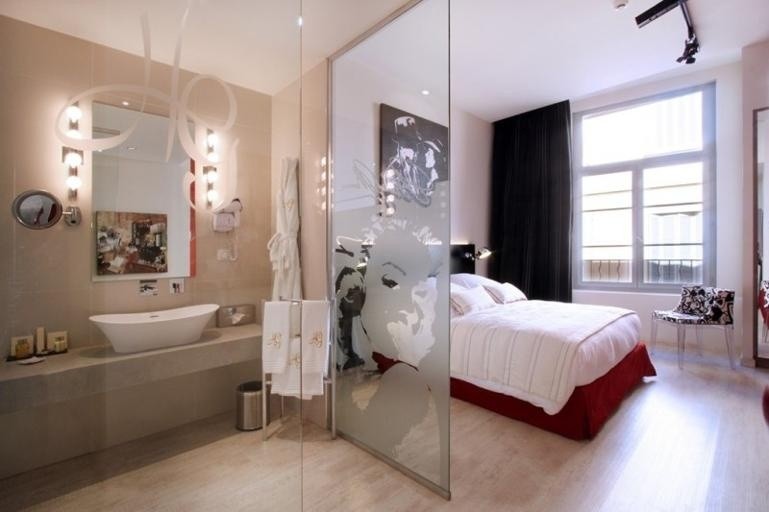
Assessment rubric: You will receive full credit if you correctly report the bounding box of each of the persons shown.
[385,116,423,193]
[423,134,448,191]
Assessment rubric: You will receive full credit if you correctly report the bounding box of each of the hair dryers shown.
[212,197,243,263]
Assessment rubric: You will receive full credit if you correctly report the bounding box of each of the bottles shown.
[35,326,46,352]
[15,339,29,358]
[54,336,66,352]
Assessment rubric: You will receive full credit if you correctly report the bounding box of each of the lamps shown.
[207,129,215,152]
[636,0,699,63]
[466,248,491,258]
[62,147,84,199]
[203,166,219,206]
[66,103,81,130]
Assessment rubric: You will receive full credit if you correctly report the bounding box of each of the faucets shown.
[135,285,160,297]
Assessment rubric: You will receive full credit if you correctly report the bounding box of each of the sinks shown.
[88,304,220,354]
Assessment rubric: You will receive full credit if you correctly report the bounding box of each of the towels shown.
[261,301,290,374]
[301,301,329,376]
[272,338,324,400]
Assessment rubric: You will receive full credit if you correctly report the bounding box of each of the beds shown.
[336,245,657,441]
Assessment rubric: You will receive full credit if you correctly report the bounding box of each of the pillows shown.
[450,287,497,315]
[450,272,500,287]
[484,282,527,303]
[393,278,467,319]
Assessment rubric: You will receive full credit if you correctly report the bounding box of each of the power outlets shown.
[169,278,184,294]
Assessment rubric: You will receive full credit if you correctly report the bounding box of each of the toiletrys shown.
[15,338,29,358]
[54,336,65,352]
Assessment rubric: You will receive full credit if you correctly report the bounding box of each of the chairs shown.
[652,286,736,370]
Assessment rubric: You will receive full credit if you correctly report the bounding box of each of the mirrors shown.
[753,108,769,369]
[92,103,195,282]
[9,189,80,228]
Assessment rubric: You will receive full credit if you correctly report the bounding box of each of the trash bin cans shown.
[235,381,271,432]
